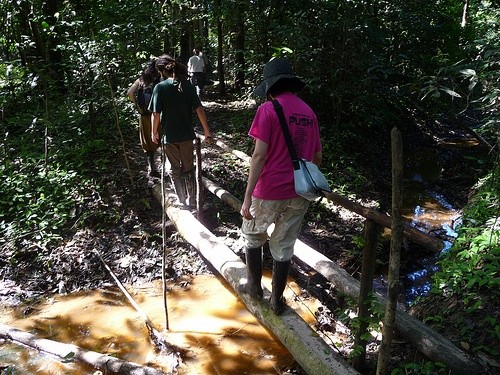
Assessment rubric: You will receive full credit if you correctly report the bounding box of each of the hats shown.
[254,60,305,98]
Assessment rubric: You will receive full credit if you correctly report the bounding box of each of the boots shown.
[271,259,291,314]
[237,246,264,298]
[184,177,197,212]
[148,154,161,178]
[168,176,188,209]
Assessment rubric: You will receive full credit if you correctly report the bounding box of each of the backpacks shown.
[135,79,154,116]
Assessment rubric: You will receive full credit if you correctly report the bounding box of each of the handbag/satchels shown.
[293,157,331,202]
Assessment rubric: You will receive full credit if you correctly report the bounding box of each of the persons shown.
[186,47,208,96]
[235,56,324,317]
[128,54,216,211]
